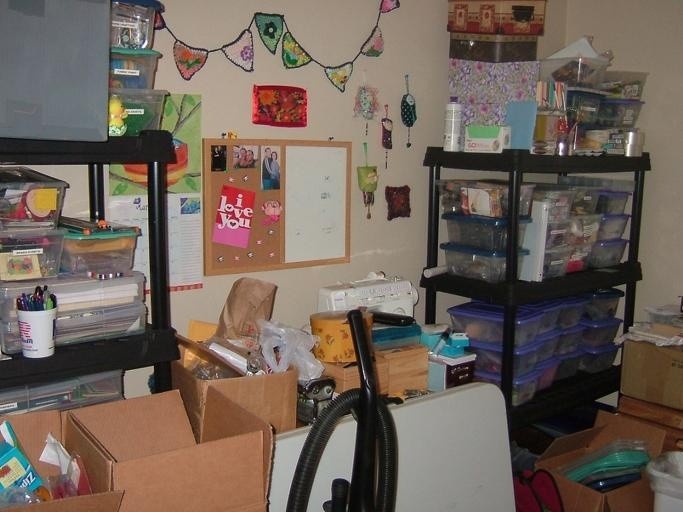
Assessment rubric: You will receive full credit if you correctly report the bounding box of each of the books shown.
[4,285,141,353]
[0,380,121,416]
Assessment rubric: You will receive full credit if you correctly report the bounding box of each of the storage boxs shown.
[172,360,299,442]
[0,166,147,417]
[322,354,390,395]
[110,49,161,89]
[0,411,125,512]
[109,88,168,136]
[375,345,428,396]
[434,0,682,512]
[61,389,274,512]
[110,0,161,49]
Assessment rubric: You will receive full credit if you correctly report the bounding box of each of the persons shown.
[235,148,280,190]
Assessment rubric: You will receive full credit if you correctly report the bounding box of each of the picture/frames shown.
[202,137,353,277]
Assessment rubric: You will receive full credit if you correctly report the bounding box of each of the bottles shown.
[556,136,568,156]
[440,96,462,152]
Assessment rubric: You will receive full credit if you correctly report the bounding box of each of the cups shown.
[621,132,646,157]
[16,307,61,359]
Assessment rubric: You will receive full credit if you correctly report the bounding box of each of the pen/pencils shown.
[14,285,55,309]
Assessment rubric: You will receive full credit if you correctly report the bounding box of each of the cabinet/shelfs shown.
[0,129,182,393]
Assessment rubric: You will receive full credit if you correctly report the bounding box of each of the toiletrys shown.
[443,95,463,151]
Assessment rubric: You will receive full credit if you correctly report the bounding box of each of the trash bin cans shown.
[648,452,683,511]
